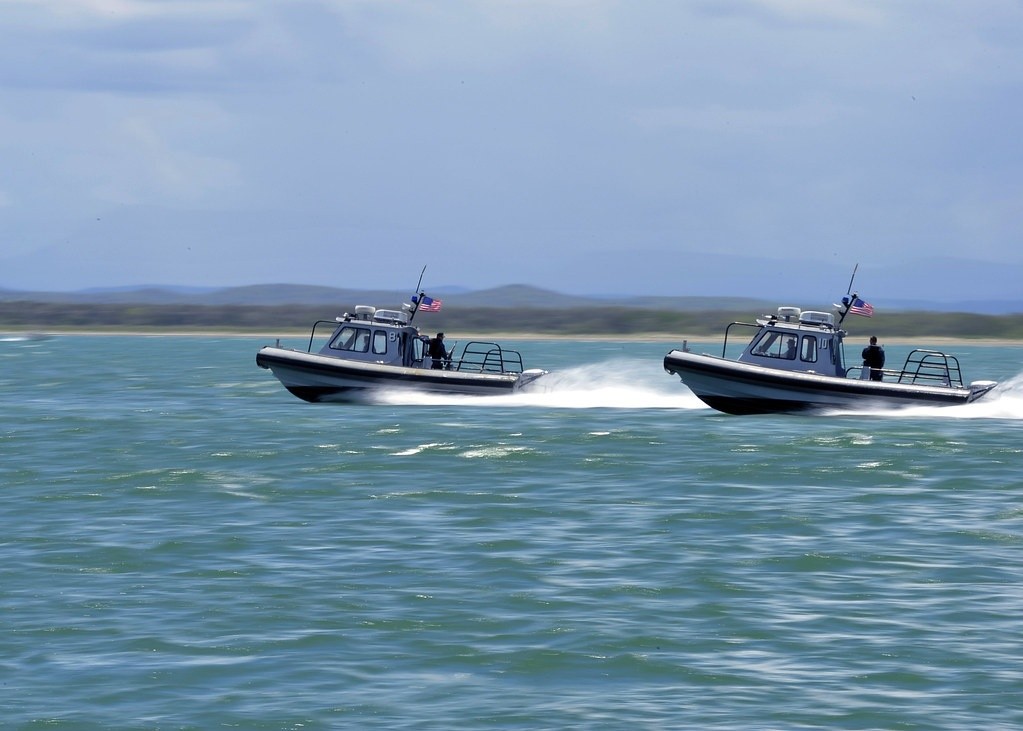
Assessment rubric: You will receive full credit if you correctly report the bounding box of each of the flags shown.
[849,298,874,318]
[418,296,441,313]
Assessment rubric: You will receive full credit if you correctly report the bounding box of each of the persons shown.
[418,332,447,370]
[862,336,885,382]
[363,336,376,354]
[770,339,796,360]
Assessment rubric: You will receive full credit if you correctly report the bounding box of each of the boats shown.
[254,263,551,405]
[661,262,1000,416]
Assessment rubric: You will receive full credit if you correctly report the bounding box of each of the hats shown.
[786,339,795,344]
[437,332,444,337]
